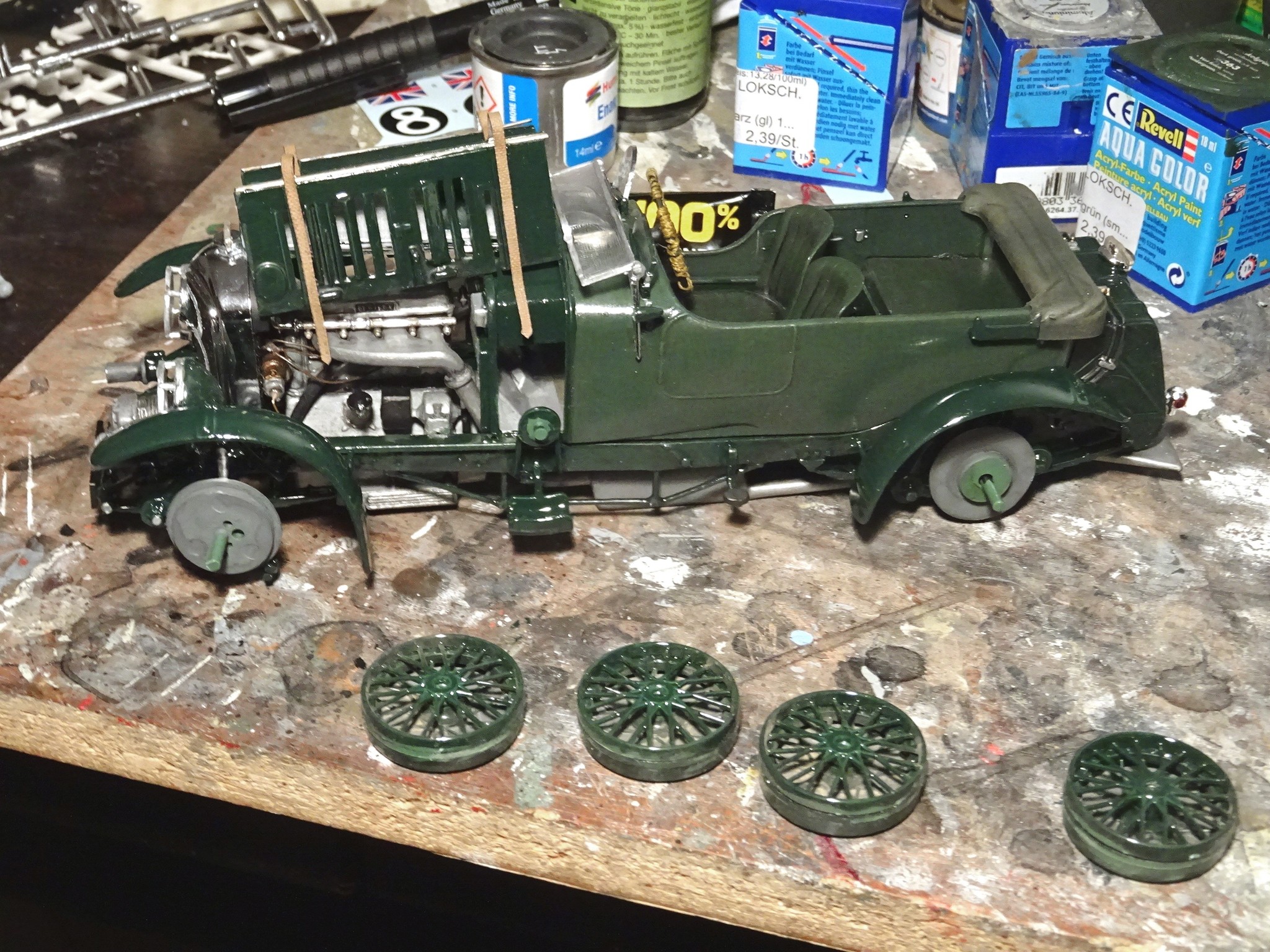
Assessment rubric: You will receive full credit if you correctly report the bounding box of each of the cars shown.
[87,117,1171,579]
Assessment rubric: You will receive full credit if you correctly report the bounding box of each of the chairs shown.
[692,199,868,323]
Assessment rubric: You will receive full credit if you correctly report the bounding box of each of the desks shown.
[0,0,1270,952]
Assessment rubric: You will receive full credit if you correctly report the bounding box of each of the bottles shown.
[559,0,715,133]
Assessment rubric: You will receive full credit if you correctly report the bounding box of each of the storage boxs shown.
[1075,19,1270,314]
[732,0,923,194]
[949,0,1128,229]
